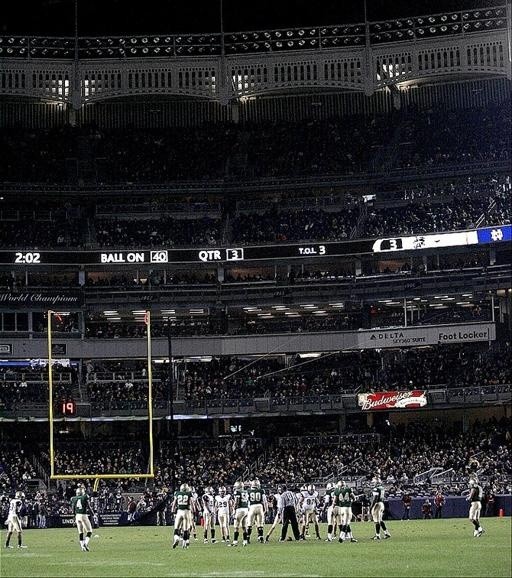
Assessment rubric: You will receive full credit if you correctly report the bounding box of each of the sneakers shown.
[171,538,180,549]
[3,544,14,549]
[471,529,486,538]
[383,533,391,540]
[16,543,27,549]
[80,543,90,553]
[370,533,382,541]
[181,531,358,549]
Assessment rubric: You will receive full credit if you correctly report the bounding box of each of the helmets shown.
[176,476,263,496]
[370,476,382,485]
[468,477,478,487]
[14,491,25,500]
[299,479,347,492]
[277,483,287,495]
[76,487,85,496]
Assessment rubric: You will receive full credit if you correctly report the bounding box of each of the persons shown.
[0,418,511,528]
[73,488,98,551]
[4,256,509,338]
[5,491,27,548]
[173,481,358,549]
[467,479,484,537]
[2,339,510,409]
[2,107,510,249]
[370,477,390,539]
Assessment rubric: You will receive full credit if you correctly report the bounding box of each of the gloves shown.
[466,494,473,503]
[163,502,377,521]
[92,515,99,525]
